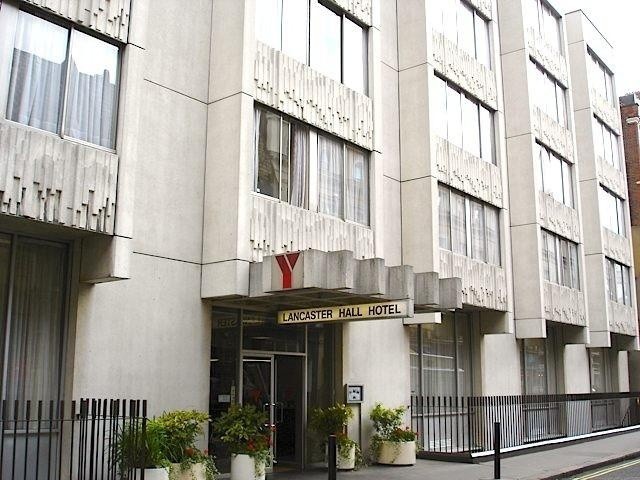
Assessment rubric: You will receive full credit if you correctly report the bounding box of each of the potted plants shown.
[306,403,368,470]
[110,416,169,480]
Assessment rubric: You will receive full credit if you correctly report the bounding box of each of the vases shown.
[231,453,266,479]
[374,440,416,467]
[171,461,207,480]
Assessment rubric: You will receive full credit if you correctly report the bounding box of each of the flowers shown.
[211,403,277,478]
[370,401,417,463]
[160,408,221,480]
